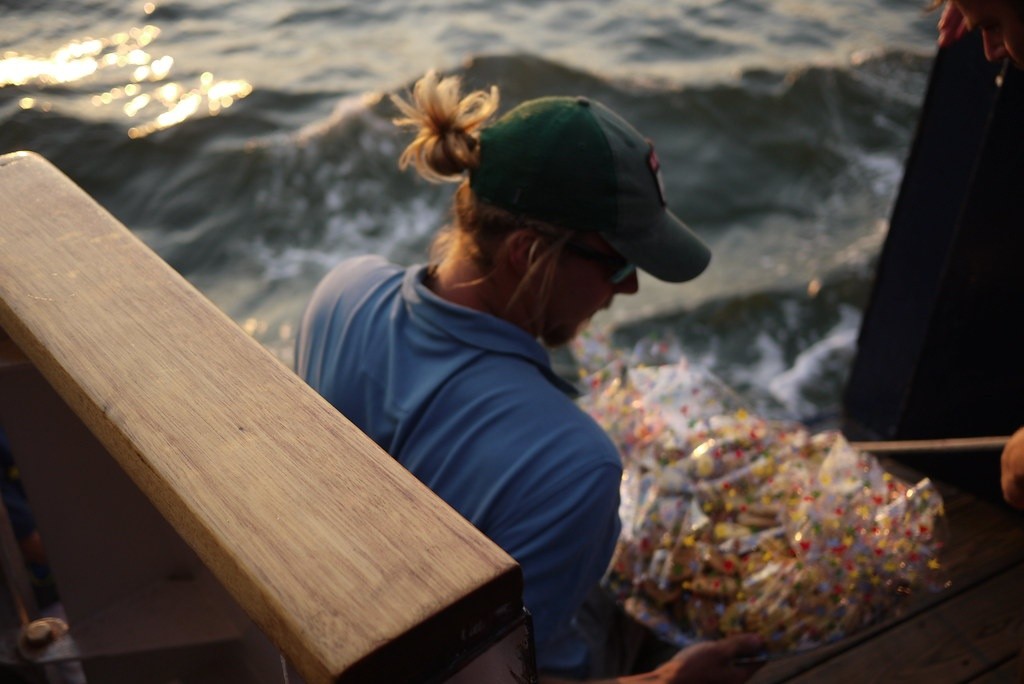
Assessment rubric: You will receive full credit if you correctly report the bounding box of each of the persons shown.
[292,69,768,684]
[924,0,1024,512]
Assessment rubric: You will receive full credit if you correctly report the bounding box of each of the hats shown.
[469,96,711,283]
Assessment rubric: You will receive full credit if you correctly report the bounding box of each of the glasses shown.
[524,224,640,295]
